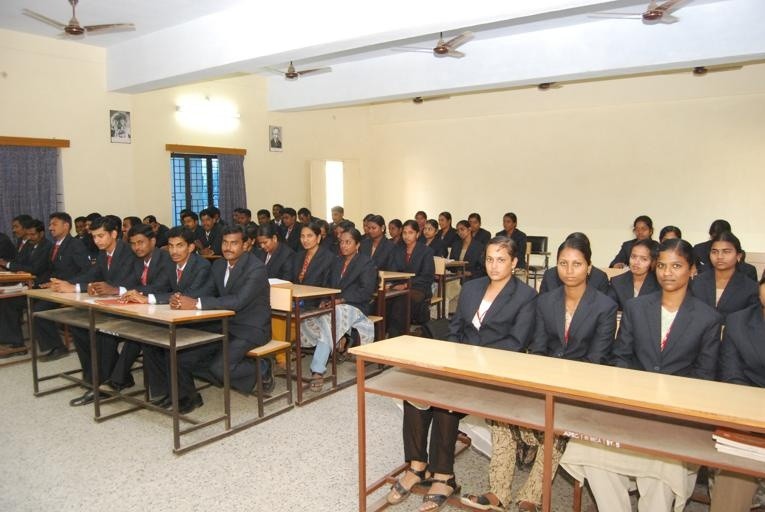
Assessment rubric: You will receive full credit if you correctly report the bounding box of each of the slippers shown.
[335,336,353,364]
[310,380,322,391]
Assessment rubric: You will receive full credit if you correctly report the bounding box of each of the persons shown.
[270,127,282,149]
[559,239,723,511]
[0,202,527,415]
[386,235,538,512]
[460,239,619,512]
[539,215,765,326]
[709,269,765,512]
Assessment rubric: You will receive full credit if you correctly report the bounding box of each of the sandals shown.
[460,492,508,511]
[386,464,433,505]
[417,475,461,512]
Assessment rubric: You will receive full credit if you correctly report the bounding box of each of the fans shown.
[21,1,134,35]
[595,0,691,23]
[265,62,331,79]
[399,32,471,58]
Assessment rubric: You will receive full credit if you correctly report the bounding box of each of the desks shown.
[348,334,760,511]
[23,286,237,448]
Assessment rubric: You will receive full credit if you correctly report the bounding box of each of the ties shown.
[19,242,183,286]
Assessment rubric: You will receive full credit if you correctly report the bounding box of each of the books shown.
[711,425,765,464]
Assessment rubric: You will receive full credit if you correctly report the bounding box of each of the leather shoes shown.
[0,343,274,415]
